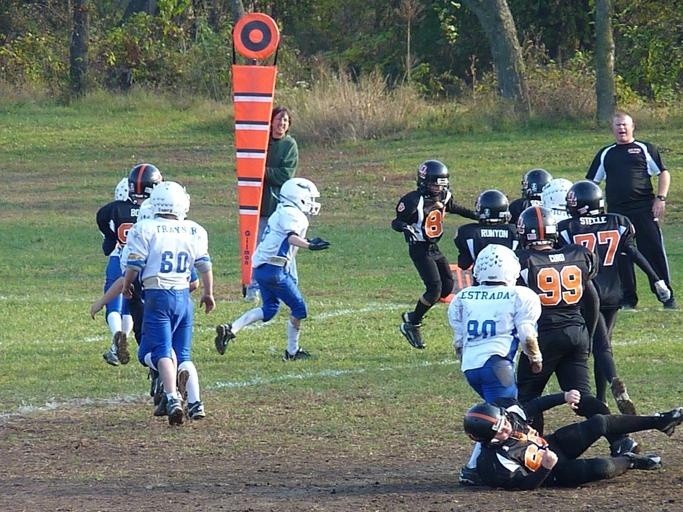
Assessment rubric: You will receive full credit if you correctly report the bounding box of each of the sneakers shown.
[610,437,642,459]
[625,448,662,471]
[283,347,313,362]
[608,377,636,414]
[666,297,677,308]
[659,408,683,436]
[112,331,131,364]
[103,345,119,366]
[215,323,235,356]
[458,465,481,486]
[399,311,427,349]
[147,368,206,425]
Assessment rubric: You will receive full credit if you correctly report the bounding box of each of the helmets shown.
[474,243,522,287]
[279,178,320,215]
[474,190,510,224]
[518,170,604,246]
[417,160,450,195]
[465,402,523,447]
[113,163,190,222]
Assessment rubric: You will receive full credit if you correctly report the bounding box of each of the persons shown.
[92,163,216,424]
[215,177,330,359]
[244,108,298,302]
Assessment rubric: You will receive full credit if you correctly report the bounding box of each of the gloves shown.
[308,237,329,251]
[403,225,421,246]
[654,280,671,303]
